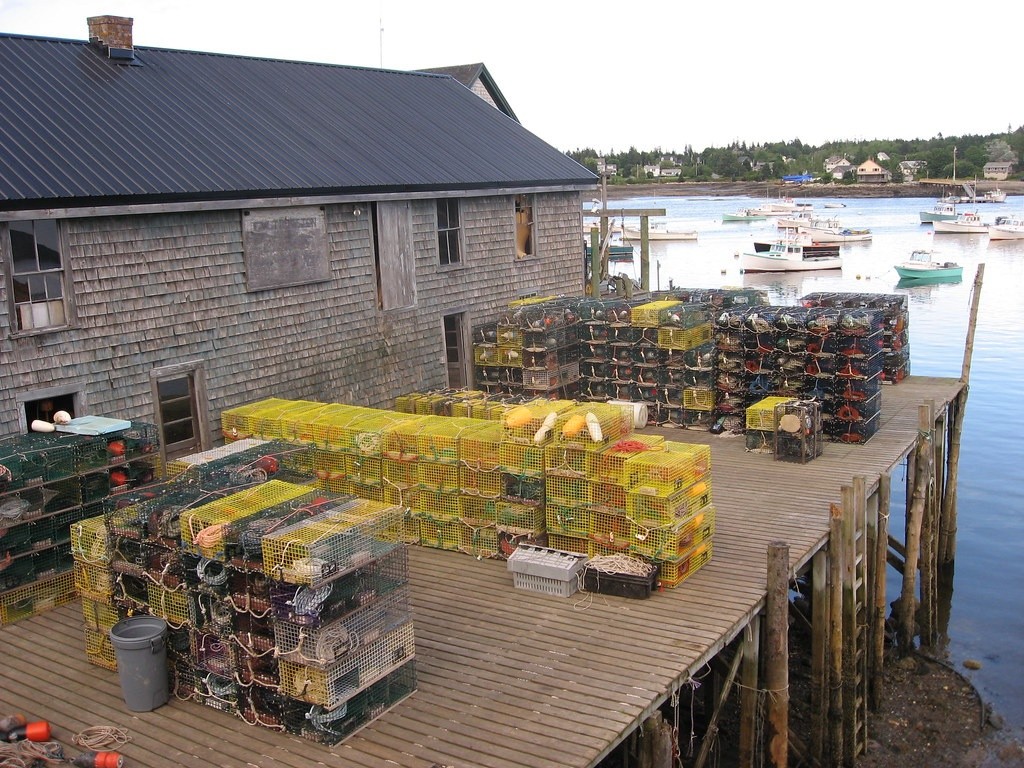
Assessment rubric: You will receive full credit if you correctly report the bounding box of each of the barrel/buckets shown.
[110,616,170,711]
[607,400,648,428]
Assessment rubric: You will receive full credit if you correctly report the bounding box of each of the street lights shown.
[954,148,957,181]
[696,162,701,175]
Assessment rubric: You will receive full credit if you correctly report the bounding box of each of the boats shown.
[894,248,962,280]
[938,194,962,204]
[583,221,634,233]
[721,184,813,221]
[932,175,990,233]
[988,215,1024,241]
[823,202,846,208]
[783,175,813,183]
[619,221,698,242]
[920,186,961,223]
[984,188,1007,203]
[740,211,873,270]
[777,211,818,228]
[587,242,635,255]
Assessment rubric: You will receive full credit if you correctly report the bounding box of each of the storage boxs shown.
[0,289,912,749]
